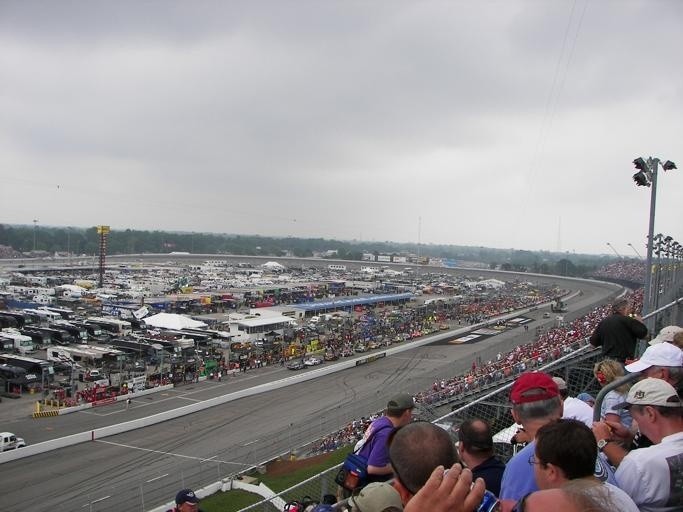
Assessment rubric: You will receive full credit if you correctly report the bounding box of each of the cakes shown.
[443,468,499,512]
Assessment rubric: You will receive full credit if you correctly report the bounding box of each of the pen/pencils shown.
[543,312,550,318]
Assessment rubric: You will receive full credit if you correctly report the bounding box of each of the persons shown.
[458,419,506,499]
[348,482,404,512]
[534,418,638,512]
[604,262,677,280]
[595,359,633,428]
[614,378,683,512]
[183,312,432,384]
[648,326,683,348]
[405,463,608,512]
[353,393,415,494]
[592,341,683,468]
[313,410,388,454]
[577,392,595,407]
[590,298,648,375]
[516,379,594,442]
[386,420,459,505]
[500,372,616,502]
[171,489,201,512]
[413,286,662,405]
[291,486,352,512]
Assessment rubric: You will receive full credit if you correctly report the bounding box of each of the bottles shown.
[612,326,683,410]
[511,372,558,404]
[387,391,420,410]
[347,482,403,512]
[175,488,200,504]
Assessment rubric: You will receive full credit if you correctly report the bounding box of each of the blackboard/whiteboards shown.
[550,295,568,313]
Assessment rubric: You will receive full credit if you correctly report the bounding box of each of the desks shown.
[631,152,678,341]
[646,232,683,314]
[31,219,38,249]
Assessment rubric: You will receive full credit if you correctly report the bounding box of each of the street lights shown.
[334,452,376,492]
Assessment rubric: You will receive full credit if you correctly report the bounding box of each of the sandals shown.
[0,307,226,392]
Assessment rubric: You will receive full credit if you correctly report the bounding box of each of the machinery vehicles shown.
[304,357,319,366]
[325,354,336,360]
[287,362,306,370]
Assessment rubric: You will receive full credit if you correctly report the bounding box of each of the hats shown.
[0,431,25,450]
[369,324,449,349]
[355,345,366,352]
[340,350,352,357]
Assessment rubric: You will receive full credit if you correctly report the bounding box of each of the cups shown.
[528,455,545,465]
[351,486,365,512]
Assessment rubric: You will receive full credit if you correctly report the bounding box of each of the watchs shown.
[597,439,613,452]
